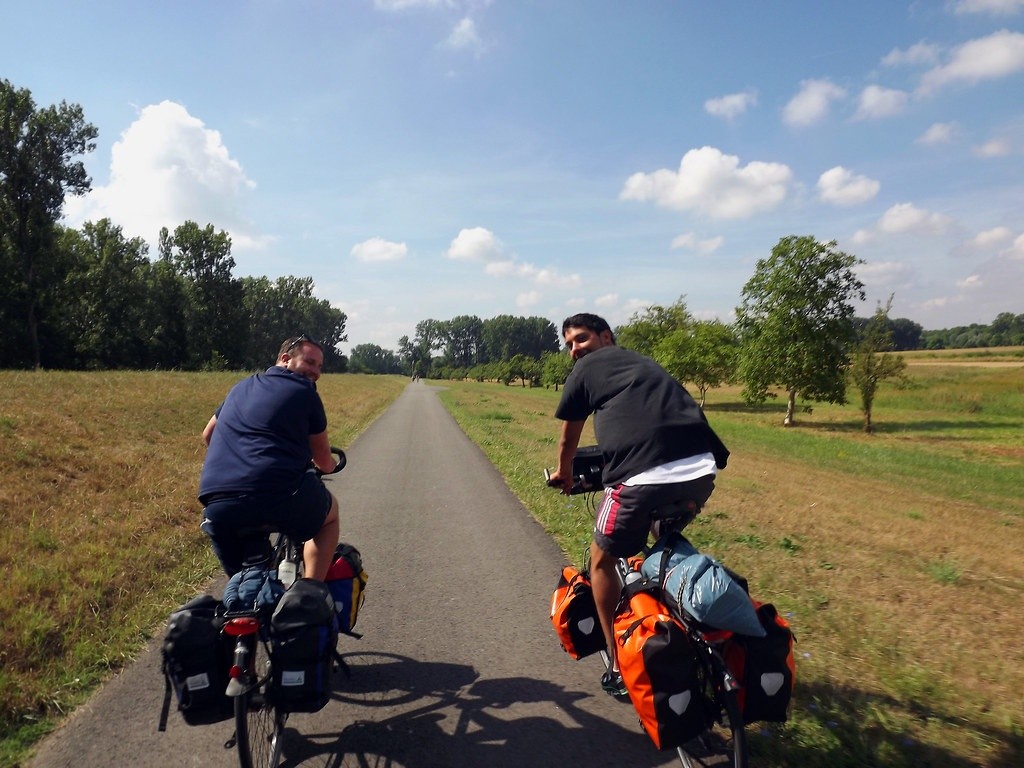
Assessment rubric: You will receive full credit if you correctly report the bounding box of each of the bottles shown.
[277,557,296,589]
[624,567,642,586]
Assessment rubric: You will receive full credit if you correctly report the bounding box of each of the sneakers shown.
[599,669,628,694]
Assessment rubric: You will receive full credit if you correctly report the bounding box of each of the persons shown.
[198,336,340,581]
[547,314,730,697]
[412,374,419,383]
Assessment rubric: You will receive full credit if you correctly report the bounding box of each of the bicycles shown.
[542,443,749,768]
[219,445,346,767]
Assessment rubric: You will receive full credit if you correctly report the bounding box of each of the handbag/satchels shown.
[699,590,797,726]
[161,594,238,725]
[550,565,608,661]
[324,540,368,633]
[264,577,338,711]
[616,578,720,752]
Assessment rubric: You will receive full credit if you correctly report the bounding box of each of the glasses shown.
[285,333,313,352]
